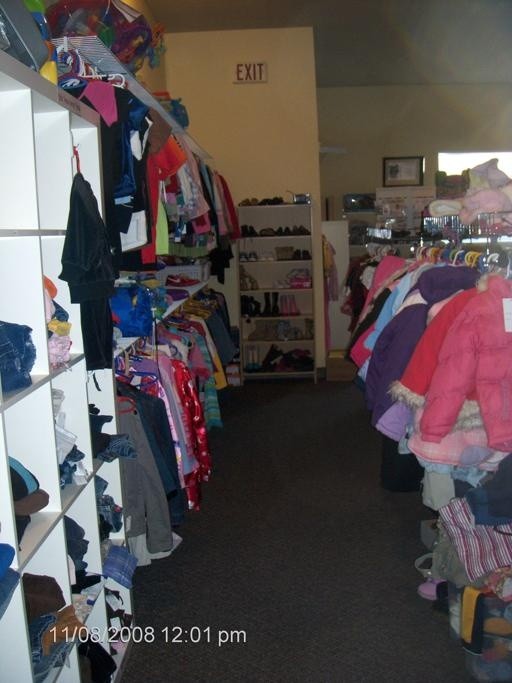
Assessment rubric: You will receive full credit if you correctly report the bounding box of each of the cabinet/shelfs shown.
[1,48,233,683]
[235,193,317,387]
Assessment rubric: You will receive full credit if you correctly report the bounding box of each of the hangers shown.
[415,246,512,280]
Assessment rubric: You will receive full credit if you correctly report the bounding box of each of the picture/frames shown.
[382,156,424,187]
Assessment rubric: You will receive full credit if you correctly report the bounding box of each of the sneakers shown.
[238,197,312,262]
[417,577,447,601]
[274,268,312,288]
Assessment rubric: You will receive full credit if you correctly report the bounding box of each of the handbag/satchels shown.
[109,276,169,338]
[240,294,261,318]
[414,540,470,589]
[239,264,258,291]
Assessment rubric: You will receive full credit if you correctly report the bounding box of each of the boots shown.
[272,292,280,316]
[262,291,271,317]
[248,318,315,341]
[280,295,289,316]
[289,295,297,316]
